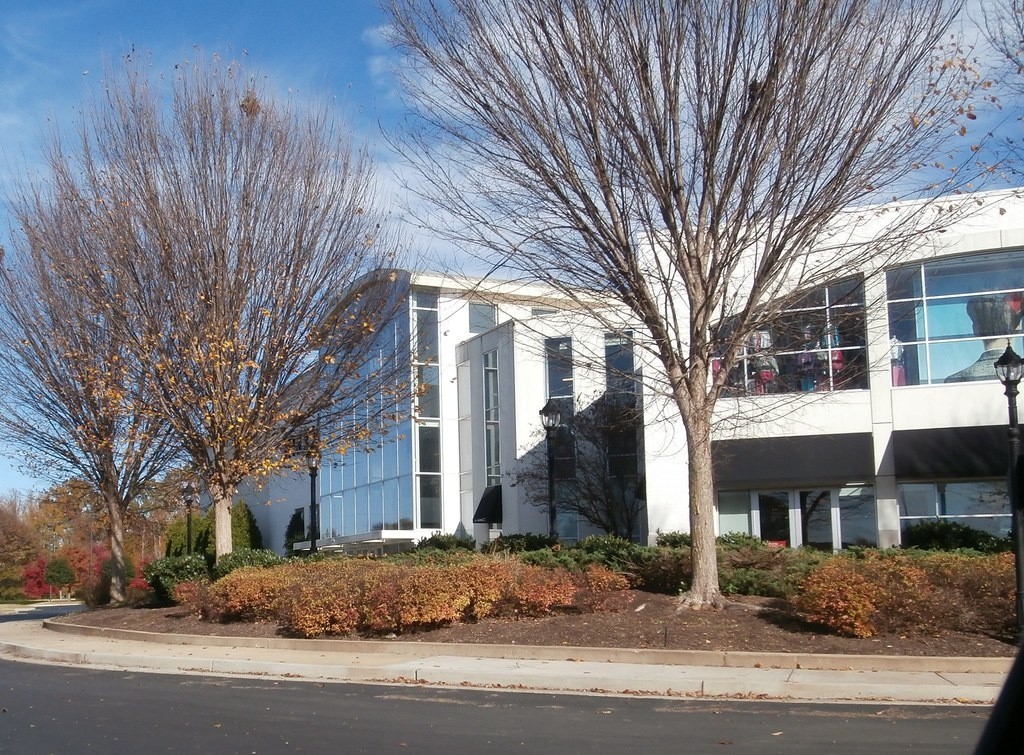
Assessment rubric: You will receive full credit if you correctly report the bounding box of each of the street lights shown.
[538,395,564,545]
[306,442,322,554]
[994,338,1024,646]
[182,483,196,554]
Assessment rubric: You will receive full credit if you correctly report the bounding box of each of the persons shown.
[712,349,779,395]
[943,296,1024,383]
[812,341,828,390]
[832,345,844,390]
[796,347,814,391]
[889,334,905,387]
